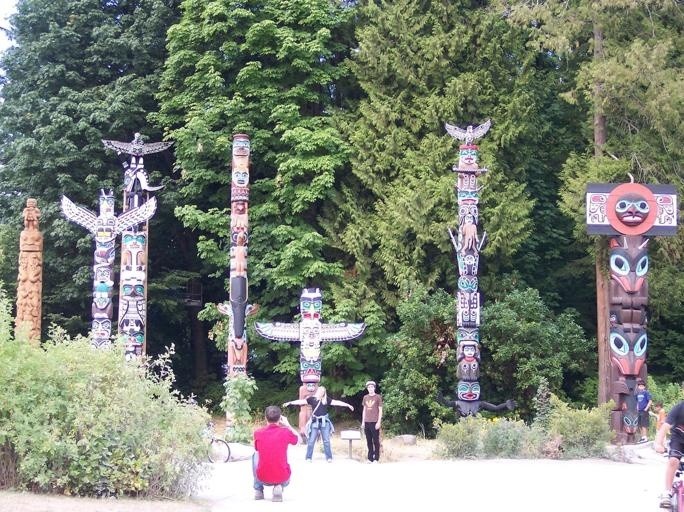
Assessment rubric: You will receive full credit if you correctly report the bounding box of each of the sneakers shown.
[256,489,264,500]
[272,485,282,502]
[660,493,673,508]
[637,439,649,444]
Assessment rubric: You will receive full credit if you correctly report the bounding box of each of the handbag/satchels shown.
[303,419,313,438]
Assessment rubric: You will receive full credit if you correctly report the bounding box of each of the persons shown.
[650,400,668,453]
[251,406,303,502]
[654,399,684,509]
[22,197,42,231]
[633,380,653,443]
[361,380,383,462]
[281,386,354,462]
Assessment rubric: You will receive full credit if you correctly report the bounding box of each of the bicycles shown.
[652,446,684,512]
[204,422,231,466]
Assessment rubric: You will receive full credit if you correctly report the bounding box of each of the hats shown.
[366,381,376,387]
